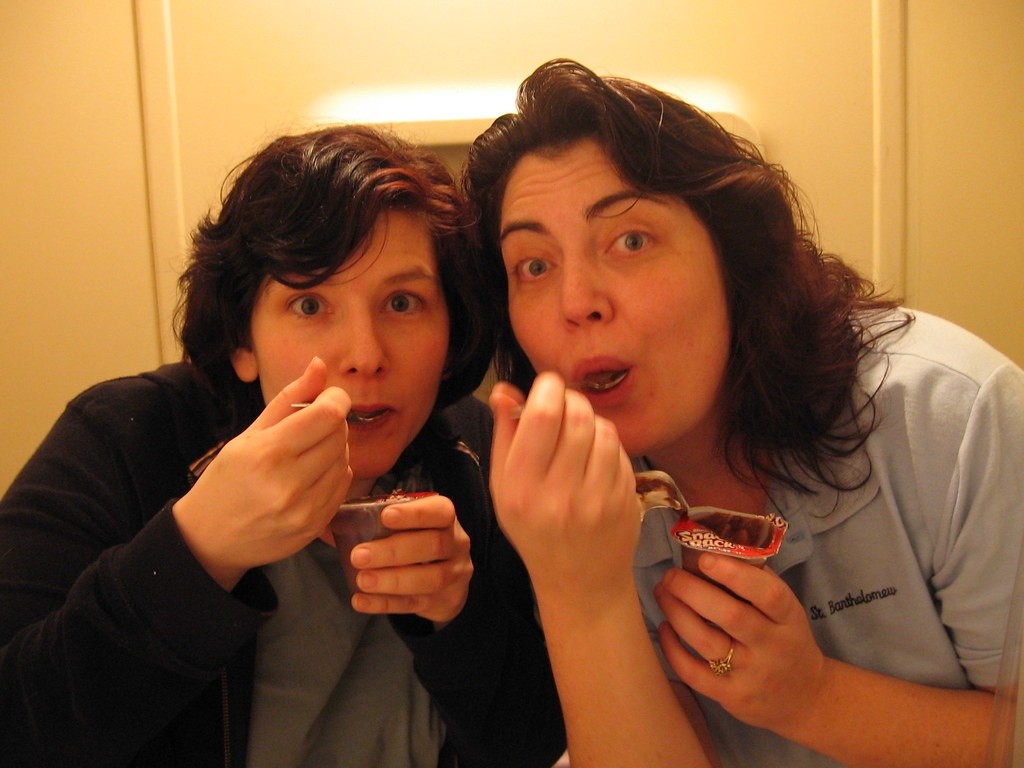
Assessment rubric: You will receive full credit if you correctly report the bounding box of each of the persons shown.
[1,124,566,768]
[464,59,1024,768]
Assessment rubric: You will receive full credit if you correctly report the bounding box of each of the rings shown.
[707,634,740,681]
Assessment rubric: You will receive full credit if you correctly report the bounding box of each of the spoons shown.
[509,369,628,420]
[291,403,389,424]
